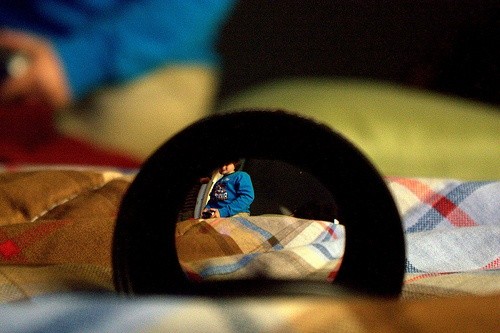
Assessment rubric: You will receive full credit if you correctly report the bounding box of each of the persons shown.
[0,1,231,172]
[203,159,255,218]
